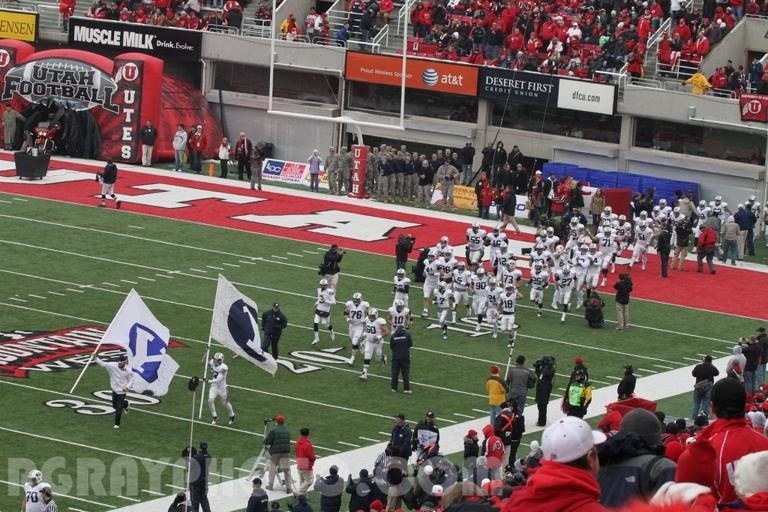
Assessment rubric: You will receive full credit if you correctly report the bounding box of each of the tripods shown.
[246,446,284,486]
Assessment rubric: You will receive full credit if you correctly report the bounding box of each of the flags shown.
[103,289,180,398]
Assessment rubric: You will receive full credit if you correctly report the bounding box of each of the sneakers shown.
[347,357,354,365]
[601,278,607,287]
[532,297,584,321]
[173,168,182,171]
[358,374,368,379]
[97,200,122,209]
[628,261,647,271]
[213,415,235,425]
[113,425,119,428]
[465,307,516,348]
[330,332,335,341]
[124,402,131,412]
[312,339,320,344]
[419,313,456,339]
[391,389,412,394]
[383,356,388,367]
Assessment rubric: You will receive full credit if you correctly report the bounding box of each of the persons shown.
[219,132,266,192]
[1,104,27,150]
[313,198,768,380]
[166,326,768,512]
[94,155,124,209]
[171,122,205,174]
[19,468,60,512]
[1,0,767,97]
[310,141,680,218]
[88,350,142,429]
[138,120,158,167]
[261,304,287,360]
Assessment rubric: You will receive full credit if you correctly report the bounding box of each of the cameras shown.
[338,250,347,256]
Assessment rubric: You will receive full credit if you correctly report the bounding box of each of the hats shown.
[273,302,279,307]
[424,466,432,475]
[518,356,525,363]
[542,416,606,464]
[431,485,444,493]
[746,384,768,412]
[663,433,715,483]
[756,327,765,331]
[738,204,745,210]
[393,414,405,419]
[39,488,51,495]
[432,150,457,161]
[622,407,662,438]
[276,416,284,424]
[427,411,434,418]
[712,378,746,412]
[468,429,478,437]
[481,478,490,486]
[489,365,500,373]
[330,144,356,153]
[368,143,428,167]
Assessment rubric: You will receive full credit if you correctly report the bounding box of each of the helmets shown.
[428,222,523,293]
[28,470,42,486]
[640,199,680,229]
[748,194,756,203]
[395,300,404,311]
[728,216,735,222]
[699,200,707,210]
[534,206,626,273]
[368,308,378,318]
[397,268,405,276]
[715,195,721,205]
[352,292,362,301]
[213,353,223,365]
[319,278,328,286]
